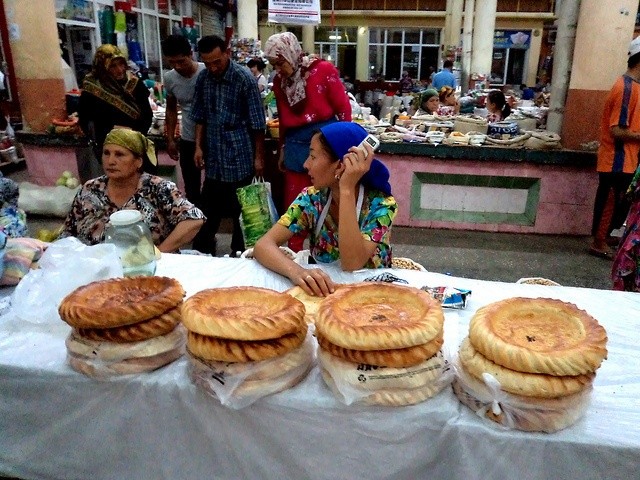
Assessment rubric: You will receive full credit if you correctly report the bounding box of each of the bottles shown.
[105,209,156,278]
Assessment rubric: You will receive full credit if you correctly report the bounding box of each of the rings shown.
[304,274,310,281]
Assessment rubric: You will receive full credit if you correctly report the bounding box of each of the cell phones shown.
[350,135,380,160]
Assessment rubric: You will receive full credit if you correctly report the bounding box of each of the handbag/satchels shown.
[238,175,280,249]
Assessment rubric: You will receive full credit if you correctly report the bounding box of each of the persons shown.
[413,89,440,116]
[262,32,353,253]
[51,128,208,254]
[431,60,458,89]
[245,58,268,94]
[126,60,163,105]
[76,44,153,167]
[254,120,399,298]
[439,85,462,115]
[589,35,640,260]
[484,90,510,126]
[519,83,534,99]
[192,35,265,259]
[162,34,207,207]
[400,72,413,88]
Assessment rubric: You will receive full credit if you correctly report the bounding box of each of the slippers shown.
[585,247,615,260]
[608,236,620,245]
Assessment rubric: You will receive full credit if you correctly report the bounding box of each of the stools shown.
[391,256,425,271]
[517,276,561,285]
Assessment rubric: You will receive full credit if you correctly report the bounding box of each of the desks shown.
[1,236,640,480]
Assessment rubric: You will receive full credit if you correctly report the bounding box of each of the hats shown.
[627,35,640,62]
